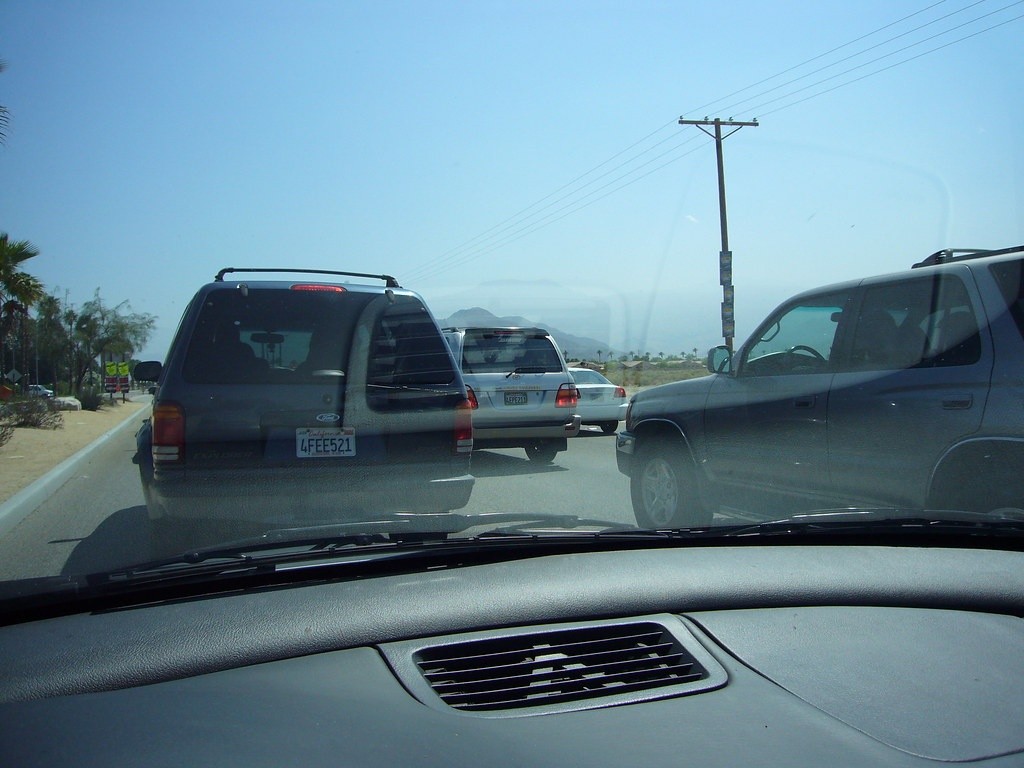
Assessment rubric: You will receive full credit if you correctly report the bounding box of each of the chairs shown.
[208,341,256,375]
[296,321,354,375]
[885,326,930,360]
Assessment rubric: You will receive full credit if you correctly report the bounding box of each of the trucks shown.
[103,364,129,392]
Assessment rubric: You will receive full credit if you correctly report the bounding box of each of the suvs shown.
[439,326,580,464]
[130,268,476,529]
[616,243,1023,531]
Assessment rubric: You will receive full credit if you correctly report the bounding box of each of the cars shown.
[568,367,629,434]
[29,384,52,400]
